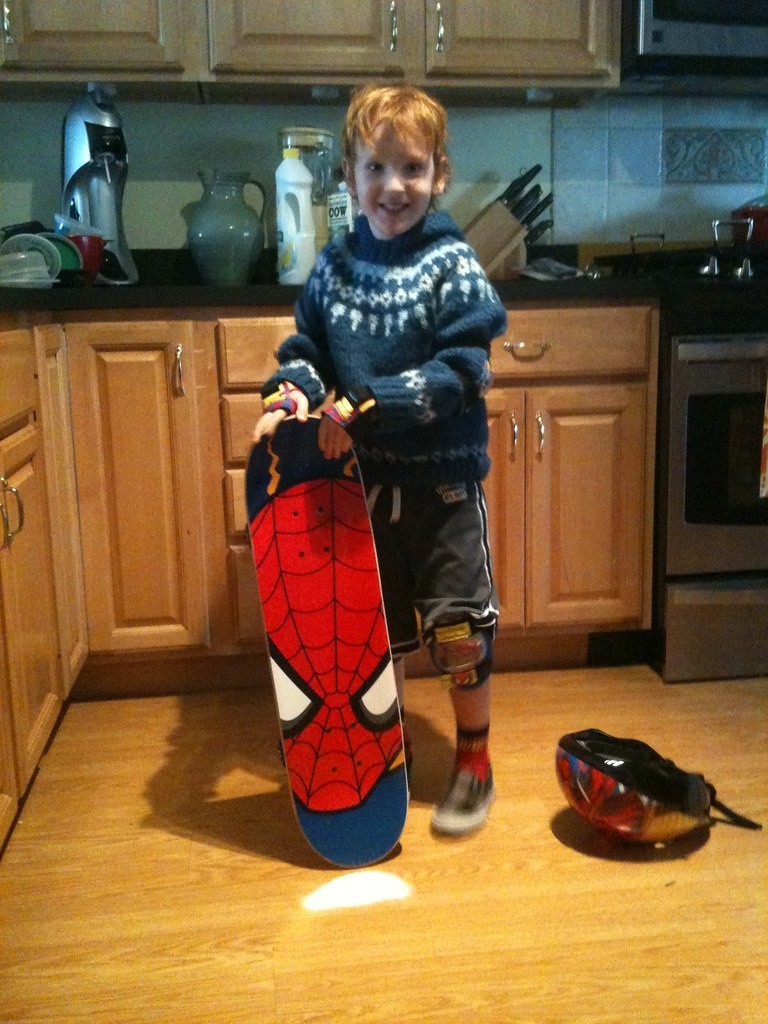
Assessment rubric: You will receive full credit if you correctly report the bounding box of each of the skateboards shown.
[245,411,404,865]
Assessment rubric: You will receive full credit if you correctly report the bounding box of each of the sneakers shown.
[430,767,495,834]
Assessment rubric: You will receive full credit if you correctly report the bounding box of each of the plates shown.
[1,234,61,279]
[39,232,83,270]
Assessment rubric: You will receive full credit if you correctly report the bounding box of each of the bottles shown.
[274,148,316,285]
[326,168,355,242]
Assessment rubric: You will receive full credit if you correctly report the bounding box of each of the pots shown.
[732,194,768,249]
[595,219,768,294]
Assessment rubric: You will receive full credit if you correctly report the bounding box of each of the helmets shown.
[554,730,716,841]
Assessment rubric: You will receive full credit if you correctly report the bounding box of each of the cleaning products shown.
[274,148,316,287]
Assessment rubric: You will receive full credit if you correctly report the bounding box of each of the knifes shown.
[496,164,553,248]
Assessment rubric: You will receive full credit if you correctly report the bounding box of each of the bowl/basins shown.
[50,270,99,301]
[54,213,102,237]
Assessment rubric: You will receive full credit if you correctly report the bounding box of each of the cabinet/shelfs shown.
[0,0,620,107]
[0,304,660,855]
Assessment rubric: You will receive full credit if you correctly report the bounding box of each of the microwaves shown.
[610,0,768,98]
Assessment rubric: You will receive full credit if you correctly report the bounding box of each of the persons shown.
[255,85,509,832]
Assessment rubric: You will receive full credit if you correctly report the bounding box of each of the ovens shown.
[653,337,768,683]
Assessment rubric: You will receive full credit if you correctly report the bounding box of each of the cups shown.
[68,236,106,280]
